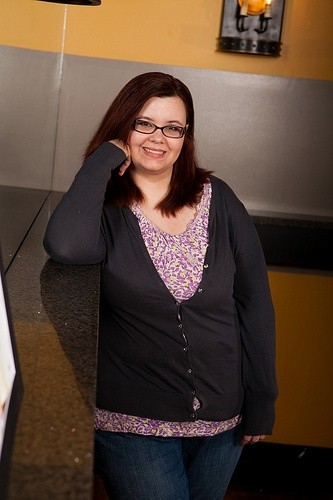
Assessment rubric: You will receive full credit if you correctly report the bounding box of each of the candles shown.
[240,0,249,17]
[263,0,272,19]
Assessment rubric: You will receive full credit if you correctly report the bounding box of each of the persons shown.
[43,72,279,500]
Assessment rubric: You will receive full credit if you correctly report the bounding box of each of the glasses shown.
[132,119,188,139]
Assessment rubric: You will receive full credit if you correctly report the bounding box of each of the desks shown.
[0,185,101,500]
